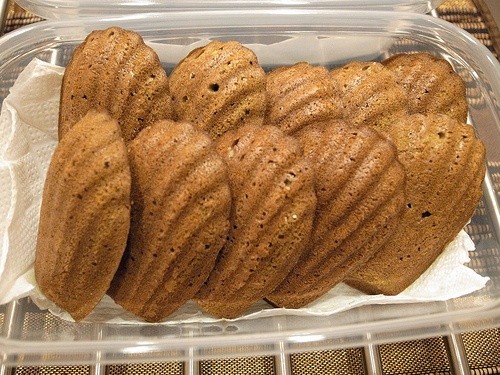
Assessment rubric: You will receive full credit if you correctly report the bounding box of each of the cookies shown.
[34,26,488,322]
